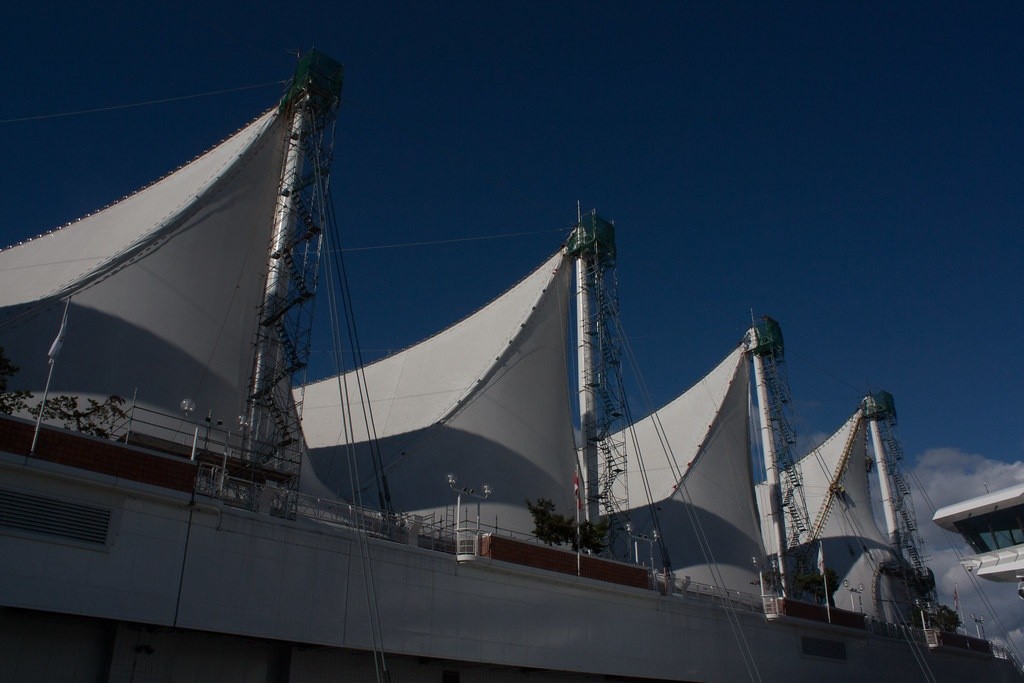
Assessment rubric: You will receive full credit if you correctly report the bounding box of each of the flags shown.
[572,468,584,512]
[47,299,70,366]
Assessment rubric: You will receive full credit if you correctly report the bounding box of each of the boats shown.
[0,69,955,627]
